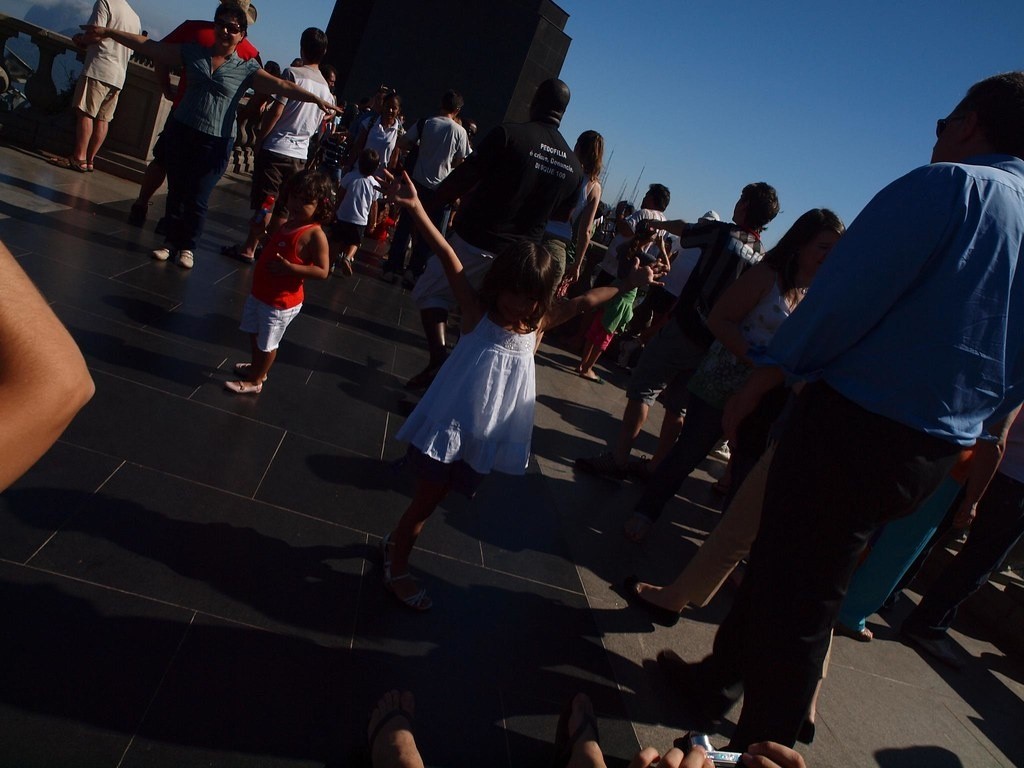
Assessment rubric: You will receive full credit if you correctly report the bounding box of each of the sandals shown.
[379,533,432,609]
[49,155,94,173]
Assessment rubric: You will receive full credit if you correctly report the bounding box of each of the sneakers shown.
[575,451,620,483]
[152,247,194,269]
[625,454,654,475]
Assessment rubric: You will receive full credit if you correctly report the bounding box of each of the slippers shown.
[575,365,604,384]
[551,691,600,768]
[221,240,263,264]
[363,696,417,768]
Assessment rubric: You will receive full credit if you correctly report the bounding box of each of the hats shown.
[698,211,717,223]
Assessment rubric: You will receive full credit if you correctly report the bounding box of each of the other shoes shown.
[833,620,875,642]
[710,446,732,461]
[901,620,961,667]
[405,356,449,389]
[224,363,268,395]
[731,569,759,595]
[342,257,353,276]
[402,271,415,291]
[131,196,149,213]
[657,650,732,722]
[622,504,654,541]
[617,573,682,628]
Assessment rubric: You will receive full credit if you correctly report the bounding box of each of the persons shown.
[0,241,96,495]
[834,403,1024,669]
[362,685,806,768]
[73,1,842,626]
[658,71,1024,753]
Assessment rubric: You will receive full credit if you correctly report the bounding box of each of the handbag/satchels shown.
[403,116,427,184]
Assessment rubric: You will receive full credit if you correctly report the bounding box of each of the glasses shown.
[935,115,962,137]
[214,18,240,34]
